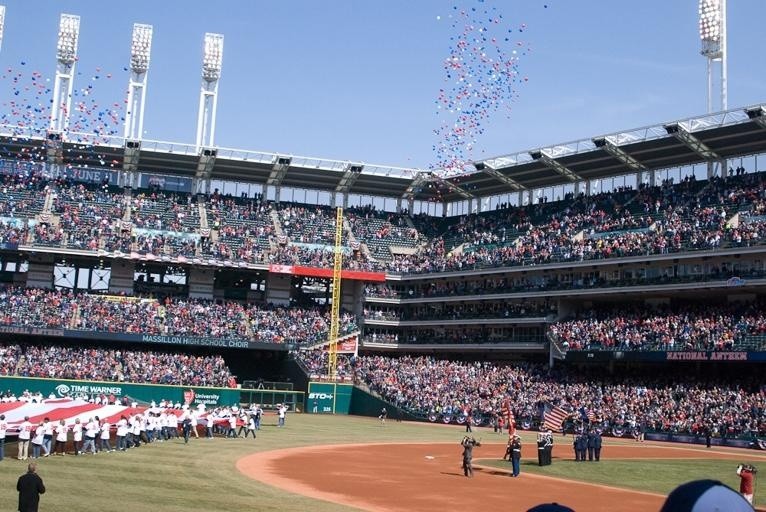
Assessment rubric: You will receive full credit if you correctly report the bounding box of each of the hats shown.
[659,479,756,512]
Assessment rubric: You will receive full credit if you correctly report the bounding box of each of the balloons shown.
[425,0,555,166]
[0,54,138,166]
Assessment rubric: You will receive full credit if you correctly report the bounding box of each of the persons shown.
[0,167,334,389]
[342,168,766,449]
[1,387,289,461]
[16,463,45,511]
[736,463,759,507]
[459,432,602,478]
